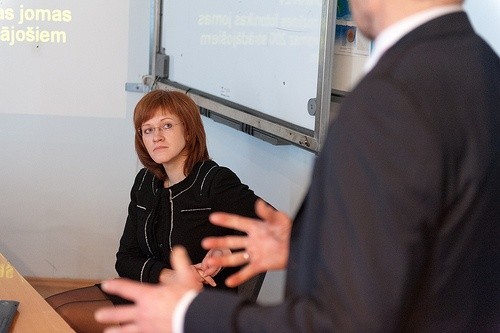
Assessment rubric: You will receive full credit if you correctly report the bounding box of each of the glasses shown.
[137,120,183,135]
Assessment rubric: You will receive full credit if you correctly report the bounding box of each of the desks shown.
[0,252,77,333]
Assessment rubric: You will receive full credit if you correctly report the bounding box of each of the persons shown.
[45,88,278,332]
[93,0,500,333]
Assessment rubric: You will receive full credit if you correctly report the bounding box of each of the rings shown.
[242,252,250,260]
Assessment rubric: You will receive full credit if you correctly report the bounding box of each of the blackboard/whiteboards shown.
[142,0,338,157]
